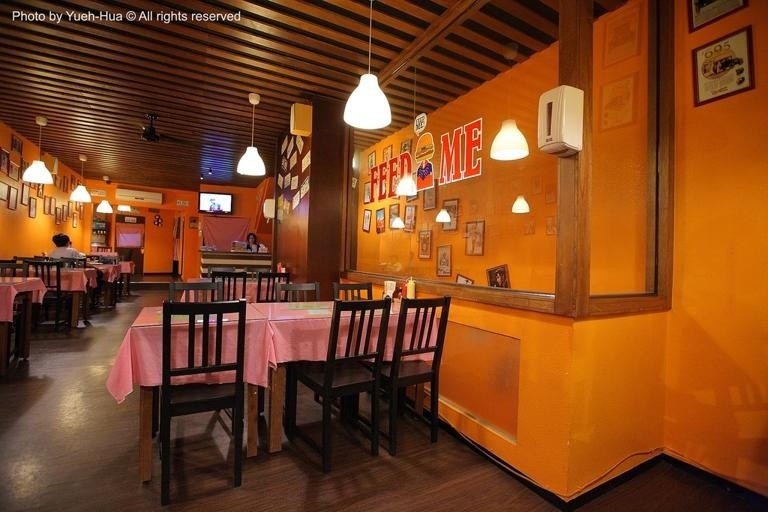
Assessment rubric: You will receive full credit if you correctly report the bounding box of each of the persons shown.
[493,268,508,288]
[50,233,85,268]
[210,199,221,211]
[247,233,260,249]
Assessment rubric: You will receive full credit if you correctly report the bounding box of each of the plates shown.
[89,263,103,265]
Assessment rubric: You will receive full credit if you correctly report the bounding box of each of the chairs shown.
[0,247,452,505]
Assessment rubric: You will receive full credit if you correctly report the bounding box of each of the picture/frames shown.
[687,0,748,33]
[0,136,84,228]
[522,175,559,237]
[692,25,755,107]
[362,138,511,289]
[599,3,643,134]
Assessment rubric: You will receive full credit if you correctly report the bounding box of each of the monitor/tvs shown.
[197,191,233,215]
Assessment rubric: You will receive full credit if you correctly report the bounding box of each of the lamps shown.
[70,154,91,203]
[344,0,391,130]
[22,117,53,184]
[491,42,530,160]
[200,168,212,179]
[96,176,114,213]
[237,92,266,177]
[290,103,312,136]
[512,195,530,213]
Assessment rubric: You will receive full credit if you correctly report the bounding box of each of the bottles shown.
[398,277,416,300]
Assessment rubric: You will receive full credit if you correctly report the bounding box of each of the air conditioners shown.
[115,190,162,204]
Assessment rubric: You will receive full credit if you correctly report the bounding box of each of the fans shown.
[124,113,186,143]
